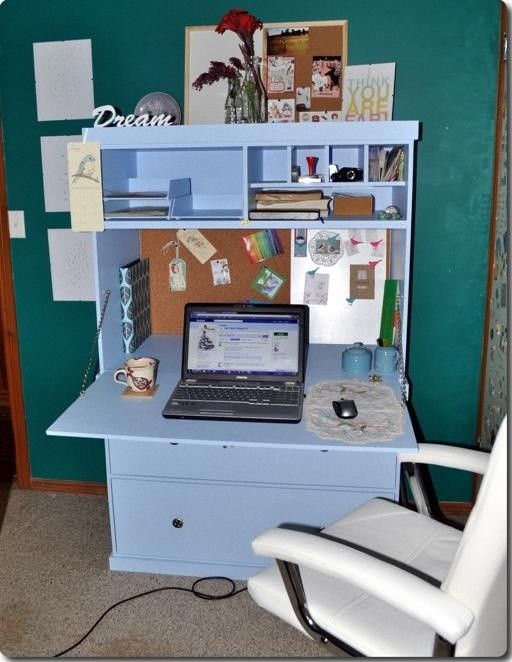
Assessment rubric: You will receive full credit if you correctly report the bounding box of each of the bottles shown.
[342,342,372,374]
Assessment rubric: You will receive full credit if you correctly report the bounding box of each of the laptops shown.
[160,302,310,424]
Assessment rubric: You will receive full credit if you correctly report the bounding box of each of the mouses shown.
[333,397,358,419]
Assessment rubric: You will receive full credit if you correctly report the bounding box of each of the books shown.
[248,189,333,221]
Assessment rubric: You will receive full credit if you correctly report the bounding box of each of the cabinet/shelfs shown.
[44,119,422,574]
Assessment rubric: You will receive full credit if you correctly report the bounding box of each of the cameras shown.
[332,167,362,182]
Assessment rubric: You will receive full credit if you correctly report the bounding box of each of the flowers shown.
[190,60,249,90]
[221,7,268,62]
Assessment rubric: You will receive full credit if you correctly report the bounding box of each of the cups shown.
[112,356,157,393]
[374,346,399,374]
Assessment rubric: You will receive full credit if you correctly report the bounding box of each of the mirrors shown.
[182,18,351,126]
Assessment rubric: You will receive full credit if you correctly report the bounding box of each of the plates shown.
[133,92,181,126]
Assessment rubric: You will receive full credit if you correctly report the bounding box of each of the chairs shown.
[247,410,512,659]
